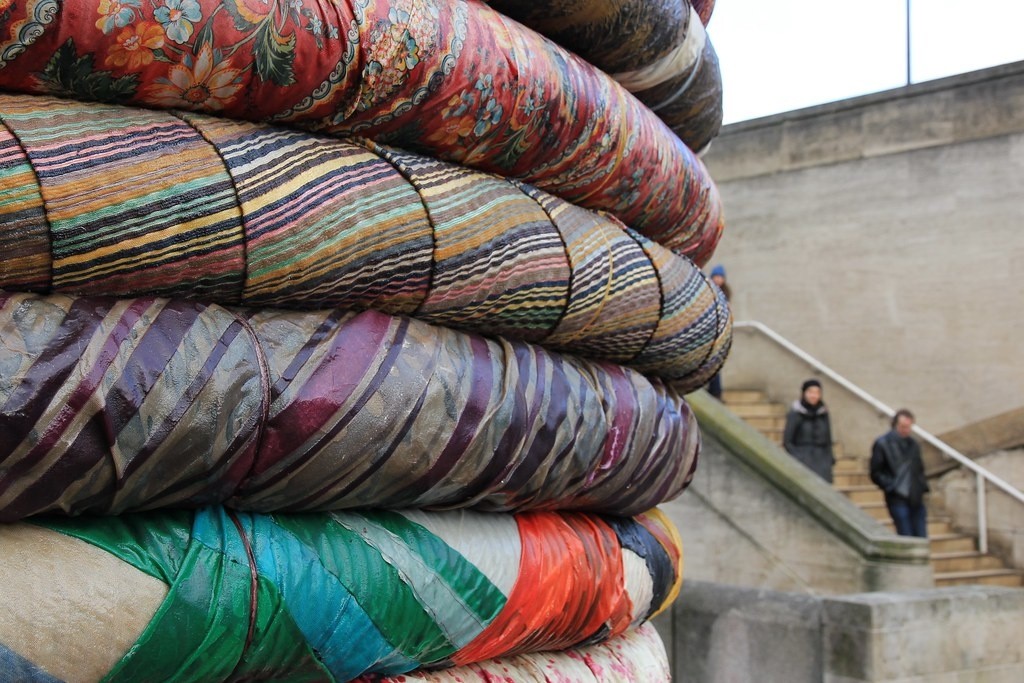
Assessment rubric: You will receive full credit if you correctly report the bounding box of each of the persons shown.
[783,380,836,484]
[708,265,733,401]
[868,409,930,536]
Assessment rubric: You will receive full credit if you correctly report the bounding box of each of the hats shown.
[803,378,820,390]
